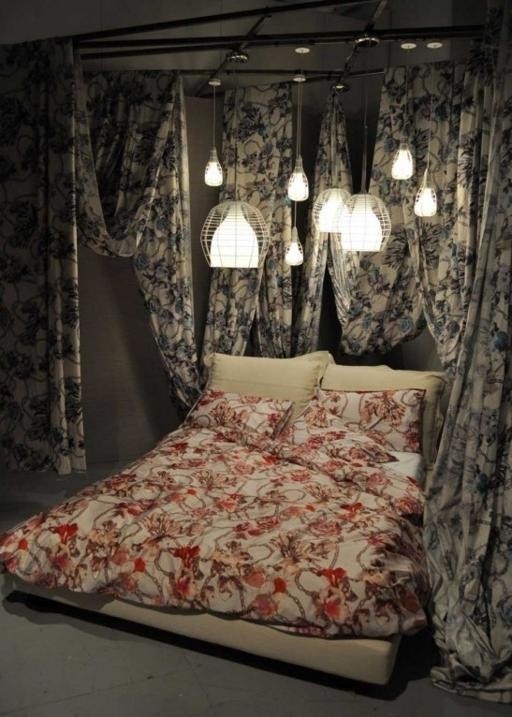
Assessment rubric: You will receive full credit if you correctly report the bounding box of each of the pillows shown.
[274,396,400,462]
[185,388,295,440]
[320,365,448,470]
[316,388,427,456]
[203,350,329,419]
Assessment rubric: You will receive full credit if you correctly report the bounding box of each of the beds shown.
[12,429,426,699]
